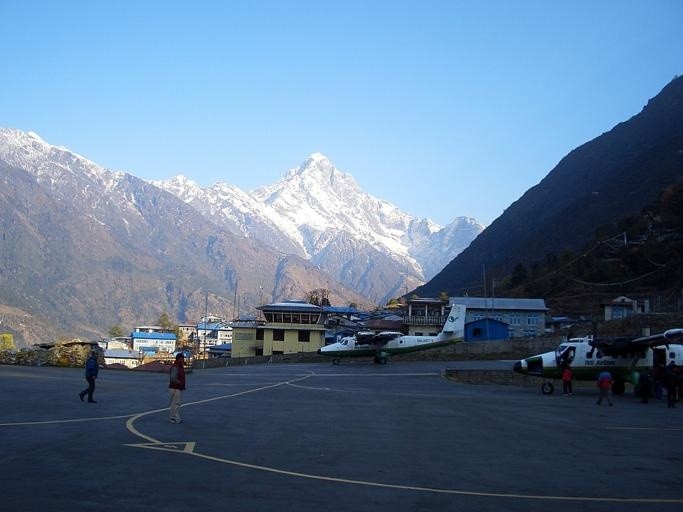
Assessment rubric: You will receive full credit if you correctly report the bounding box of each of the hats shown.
[177,353,186,358]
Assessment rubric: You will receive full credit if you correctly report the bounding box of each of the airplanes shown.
[318,303,466,365]
[512,329,682,396]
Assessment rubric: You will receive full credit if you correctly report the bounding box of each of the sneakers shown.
[169,418,184,424]
[78,393,84,402]
[88,400,96,403]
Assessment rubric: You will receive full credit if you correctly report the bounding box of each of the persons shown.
[168,353,185,423]
[631,363,683,407]
[77,352,98,402]
[560,361,573,396]
[595,367,614,406]
